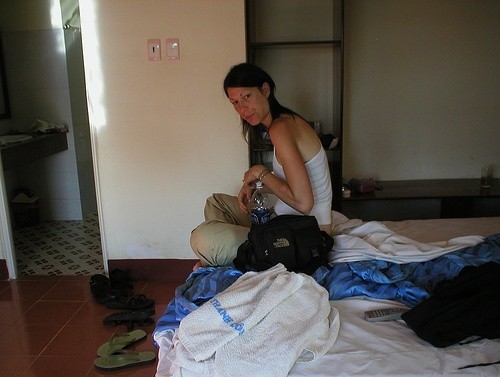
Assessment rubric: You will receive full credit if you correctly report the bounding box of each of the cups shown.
[342,186,350,197]
[480,167,493,188]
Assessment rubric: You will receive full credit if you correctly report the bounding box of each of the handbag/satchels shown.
[233,214,335,276]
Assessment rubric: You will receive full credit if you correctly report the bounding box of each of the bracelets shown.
[257,169,270,183]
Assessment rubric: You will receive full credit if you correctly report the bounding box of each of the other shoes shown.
[89,267,133,304]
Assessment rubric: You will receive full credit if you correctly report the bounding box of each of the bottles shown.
[250,181,271,228]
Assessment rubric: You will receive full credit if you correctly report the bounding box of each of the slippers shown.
[96,329,148,356]
[93,349,156,370]
[105,293,155,311]
[104,311,154,332]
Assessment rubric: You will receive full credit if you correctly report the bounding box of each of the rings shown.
[243,181,246,183]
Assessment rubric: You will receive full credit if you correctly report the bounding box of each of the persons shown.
[188,64,333,270]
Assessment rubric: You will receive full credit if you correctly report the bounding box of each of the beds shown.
[152,168,500,377]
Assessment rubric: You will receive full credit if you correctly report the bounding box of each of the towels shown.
[331,221,485,264]
[167,263,341,376]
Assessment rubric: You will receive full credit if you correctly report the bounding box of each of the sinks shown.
[0,134,32,143]
